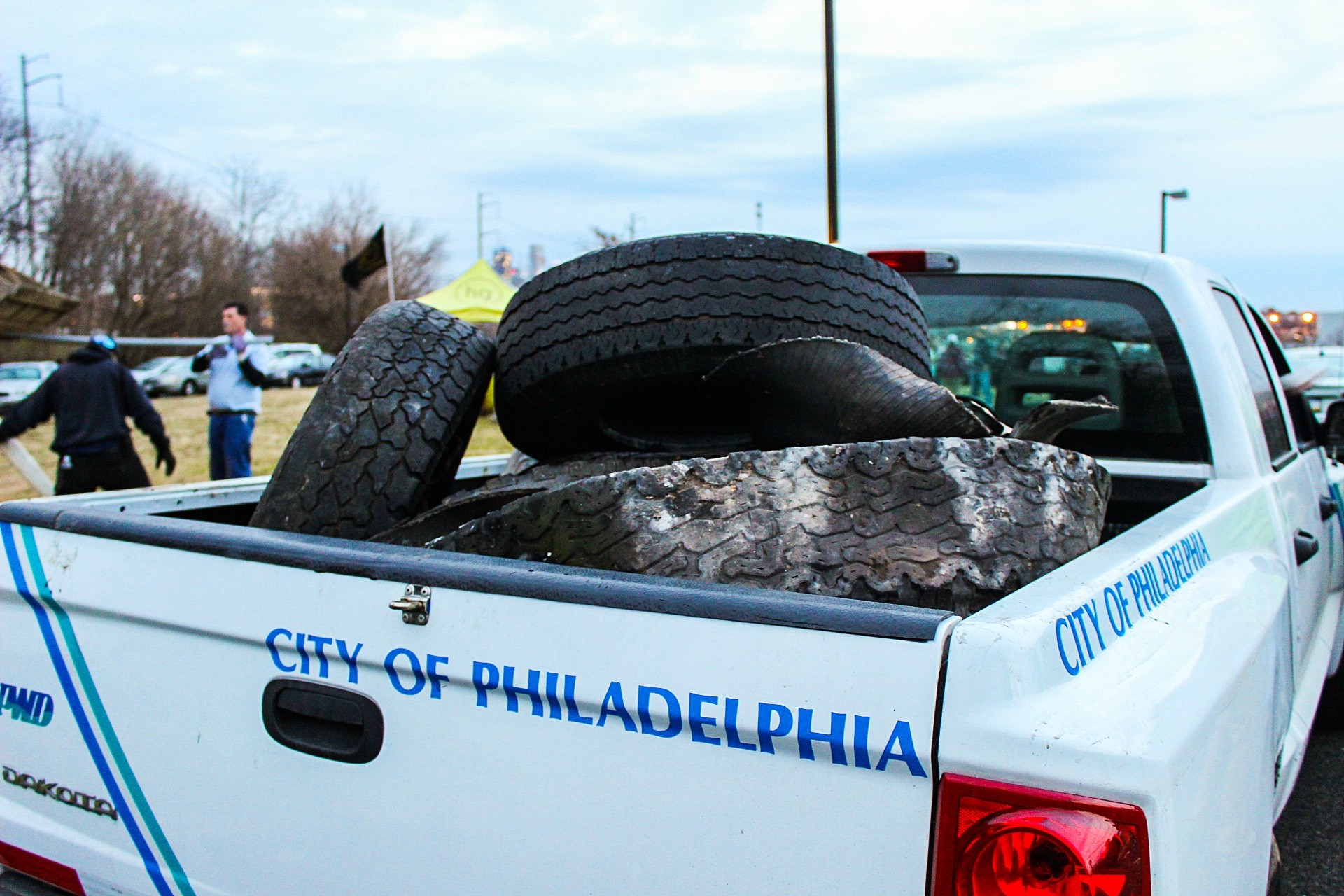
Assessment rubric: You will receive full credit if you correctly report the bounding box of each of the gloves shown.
[156,440,177,475]
[229,334,246,355]
[210,344,228,359]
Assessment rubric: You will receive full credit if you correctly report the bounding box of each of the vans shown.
[1,362,59,409]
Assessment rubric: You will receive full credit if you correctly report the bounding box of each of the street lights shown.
[1162,191,1188,252]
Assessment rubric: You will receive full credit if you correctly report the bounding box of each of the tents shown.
[414,258,518,323]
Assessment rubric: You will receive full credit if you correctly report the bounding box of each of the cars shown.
[128,341,337,397]
[1043,309,1344,451]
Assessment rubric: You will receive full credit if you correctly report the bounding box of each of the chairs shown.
[994,331,1129,434]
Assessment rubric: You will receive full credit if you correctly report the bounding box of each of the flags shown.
[342,226,387,291]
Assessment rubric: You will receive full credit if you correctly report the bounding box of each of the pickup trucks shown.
[3,245,1344,896]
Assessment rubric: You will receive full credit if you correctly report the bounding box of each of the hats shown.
[86,335,118,353]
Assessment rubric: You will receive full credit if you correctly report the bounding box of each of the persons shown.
[929,333,940,378]
[192,301,272,481]
[0,334,176,496]
[939,334,968,394]
[969,328,993,403]
